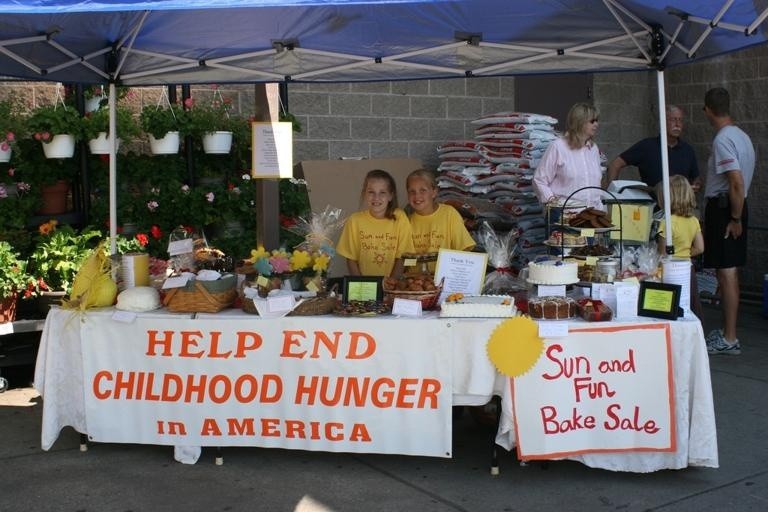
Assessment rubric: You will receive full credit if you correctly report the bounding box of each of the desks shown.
[496,303,720,478]
[33,305,512,478]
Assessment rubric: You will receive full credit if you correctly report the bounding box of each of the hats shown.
[69,243,117,314]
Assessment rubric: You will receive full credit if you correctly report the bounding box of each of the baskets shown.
[162,272,237,314]
[241,288,337,315]
[382,275,444,309]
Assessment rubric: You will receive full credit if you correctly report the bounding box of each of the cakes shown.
[577,300,613,322]
[527,259,578,282]
[528,296,576,318]
[440,294,515,317]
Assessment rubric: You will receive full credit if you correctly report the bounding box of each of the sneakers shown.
[705,329,741,355]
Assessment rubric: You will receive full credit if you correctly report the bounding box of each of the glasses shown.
[590,118,598,124]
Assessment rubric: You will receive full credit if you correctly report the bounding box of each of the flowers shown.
[197,84,253,152]
[137,98,197,144]
[0,163,310,297]
[80,105,140,143]
[0,92,27,163]
[27,102,86,146]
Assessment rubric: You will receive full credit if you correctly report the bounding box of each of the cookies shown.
[548,197,614,256]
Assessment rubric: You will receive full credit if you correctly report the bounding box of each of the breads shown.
[381,274,439,292]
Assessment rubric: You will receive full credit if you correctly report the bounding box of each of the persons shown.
[532,102,607,256]
[335,169,416,289]
[406,169,477,274]
[702,87,756,356]
[655,174,705,328]
[601,104,704,243]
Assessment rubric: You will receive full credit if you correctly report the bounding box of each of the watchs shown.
[731,216,741,223]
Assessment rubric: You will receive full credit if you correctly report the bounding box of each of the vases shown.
[89,131,120,155]
[42,134,76,158]
[148,131,180,154]
[0,296,17,323]
[202,131,232,155]
[0,142,12,162]
